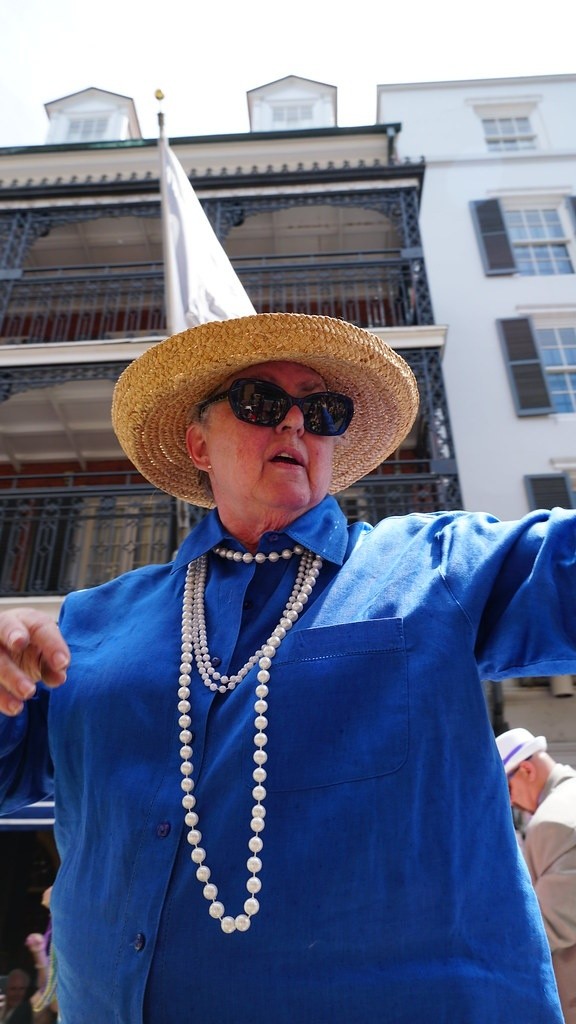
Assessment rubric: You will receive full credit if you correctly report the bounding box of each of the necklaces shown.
[30,929,56,1012]
[176,543,323,932]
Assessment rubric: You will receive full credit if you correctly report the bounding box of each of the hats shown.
[111,313,420,509]
[494,728,547,772]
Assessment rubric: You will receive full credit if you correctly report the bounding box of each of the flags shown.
[157,138,257,336]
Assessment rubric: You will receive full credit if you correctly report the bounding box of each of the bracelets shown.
[34,963,47,970]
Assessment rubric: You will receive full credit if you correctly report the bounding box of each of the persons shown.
[0,314,576,1024]
[320,401,336,434]
[0,965,55,1024]
[25,885,57,1012]
[495,728,576,1024]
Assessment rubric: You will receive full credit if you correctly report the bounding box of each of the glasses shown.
[199,378,354,436]
